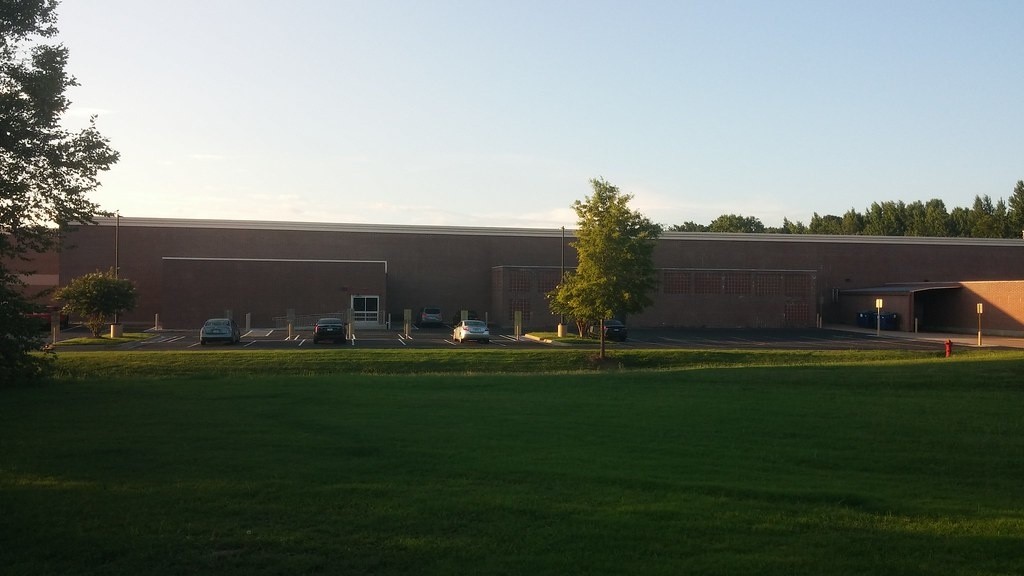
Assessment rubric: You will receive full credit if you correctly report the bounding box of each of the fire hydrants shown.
[944,340,954,357]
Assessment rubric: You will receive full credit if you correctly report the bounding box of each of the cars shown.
[416,308,443,327]
[312,318,348,343]
[453,320,491,344]
[199,318,240,345]
[26,306,69,329]
[586,318,627,342]
